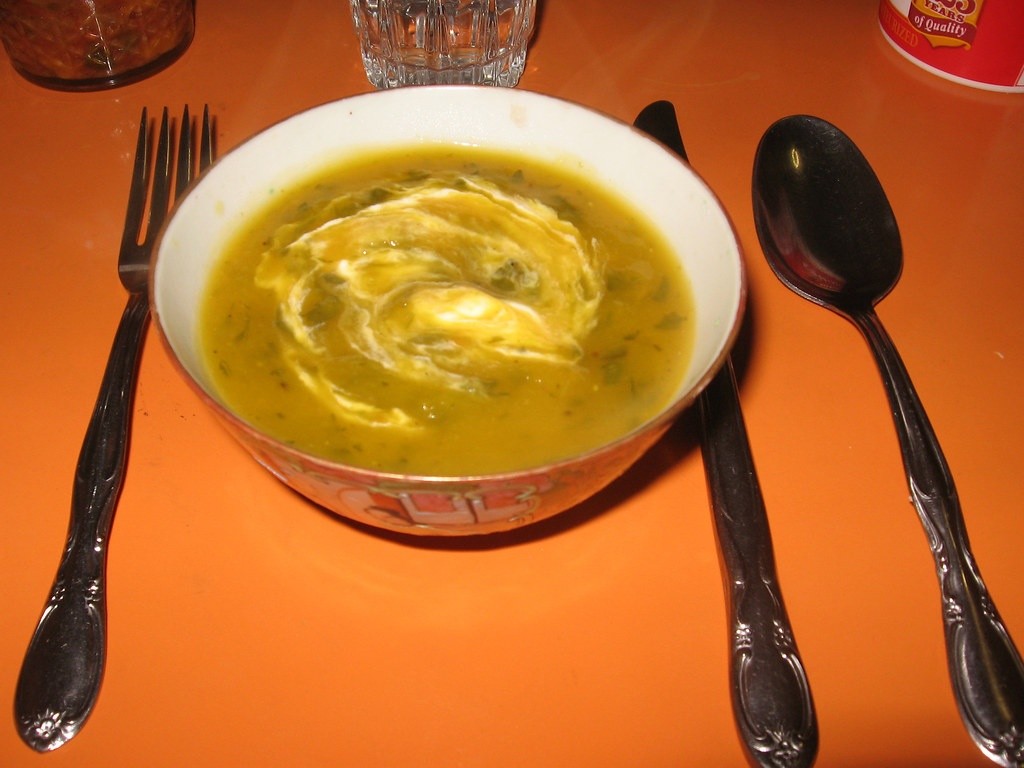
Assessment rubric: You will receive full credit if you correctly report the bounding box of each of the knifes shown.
[631,97,820,768]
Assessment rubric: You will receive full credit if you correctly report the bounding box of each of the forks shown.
[12,102,209,754]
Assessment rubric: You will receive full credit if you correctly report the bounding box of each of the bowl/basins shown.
[147,85,750,540]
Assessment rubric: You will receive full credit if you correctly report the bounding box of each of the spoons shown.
[750,113,1024,768]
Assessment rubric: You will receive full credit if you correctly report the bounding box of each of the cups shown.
[875,0,1024,94]
[1,0,196,94]
[350,0,538,90]
